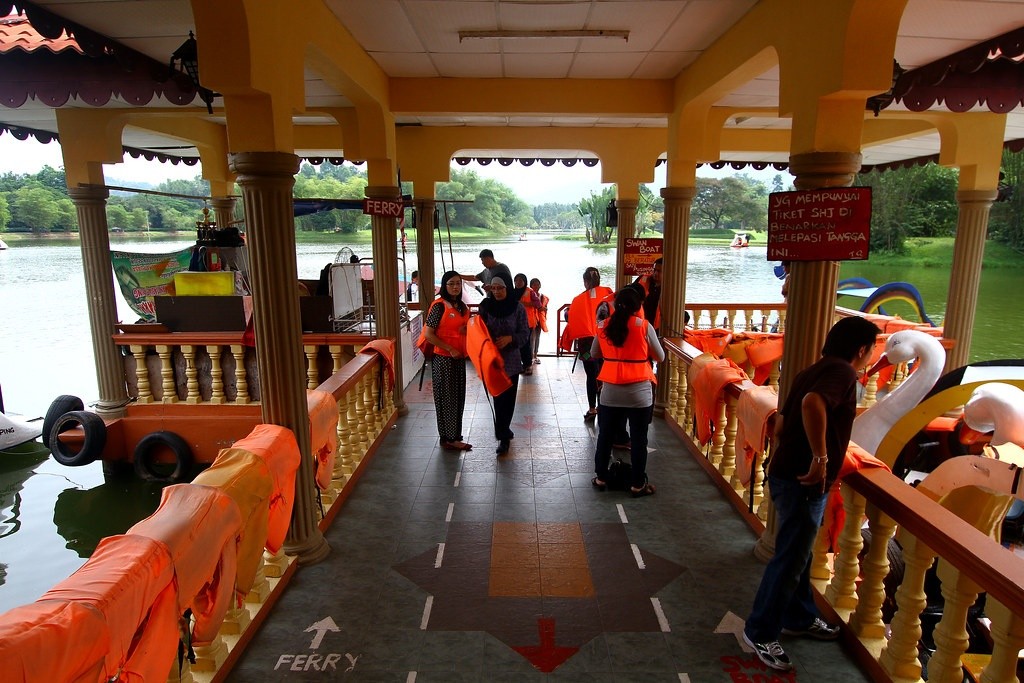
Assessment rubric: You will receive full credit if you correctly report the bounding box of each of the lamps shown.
[457,29,630,40]
[867,57,904,117]
[170,30,222,114]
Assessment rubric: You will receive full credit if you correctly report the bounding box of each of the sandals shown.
[591,477,607,491]
[631,485,657,497]
[525,365,534,376]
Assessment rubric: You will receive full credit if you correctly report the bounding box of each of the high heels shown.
[584,411,597,427]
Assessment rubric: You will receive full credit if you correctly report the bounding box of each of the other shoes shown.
[532,357,541,364]
[439,436,472,450]
[495,439,511,455]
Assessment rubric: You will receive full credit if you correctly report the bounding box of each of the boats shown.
[772,262,942,327]
[361,264,486,311]
[518,233,527,241]
[849,329,1024,683]
[397,233,409,242]
[729,234,751,247]
[0,410,45,454]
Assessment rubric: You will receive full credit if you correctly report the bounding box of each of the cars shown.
[111,227,122,232]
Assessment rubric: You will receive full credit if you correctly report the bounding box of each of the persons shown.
[564,267,614,419]
[406,271,419,302]
[478,270,530,459]
[633,258,664,340]
[513,273,550,376]
[742,315,883,673]
[684,310,690,326]
[589,282,665,496]
[737,237,742,245]
[416,270,474,453]
[458,248,515,297]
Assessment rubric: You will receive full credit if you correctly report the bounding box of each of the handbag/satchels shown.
[608,458,633,491]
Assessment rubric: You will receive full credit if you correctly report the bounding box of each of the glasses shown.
[491,286,504,291]
[446,282,461,286]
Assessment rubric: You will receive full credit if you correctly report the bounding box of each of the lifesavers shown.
[133,432,190,484]
[50,412,105,466]
[42,395,83,448]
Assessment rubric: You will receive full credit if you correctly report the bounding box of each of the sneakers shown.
[742,627,794,671]
[781,619,842,641]
